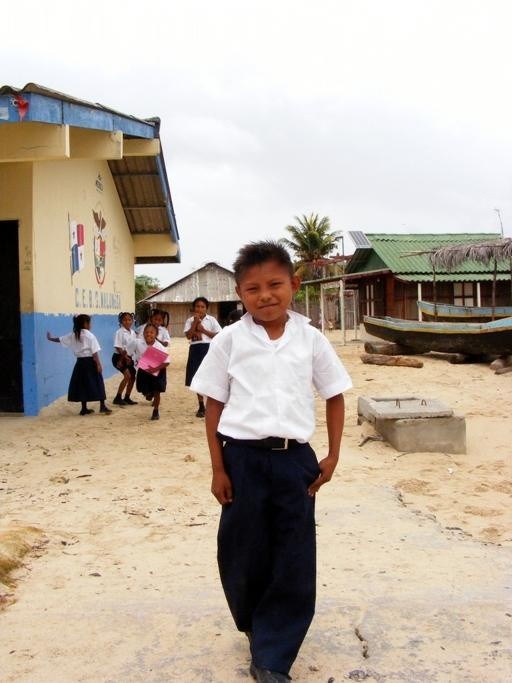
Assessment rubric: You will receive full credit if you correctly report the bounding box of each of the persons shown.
[183,296,222,417]
[46,314,113,415]
[187,240,355,683]
[111,309,171,421]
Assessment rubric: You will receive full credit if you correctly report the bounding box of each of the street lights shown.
[335,236,345,290]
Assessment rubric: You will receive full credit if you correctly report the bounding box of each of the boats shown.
[416,300,512,323]
[362,314,512,356]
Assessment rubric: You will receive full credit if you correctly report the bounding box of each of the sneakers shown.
[251,661,290,682]
[196,408,205,417]
[113,399,137,404]
[151,411,159,420]
[100,406,112,413]
[80,409,94,415]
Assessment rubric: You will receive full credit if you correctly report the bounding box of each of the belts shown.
[227,438,304,451]
